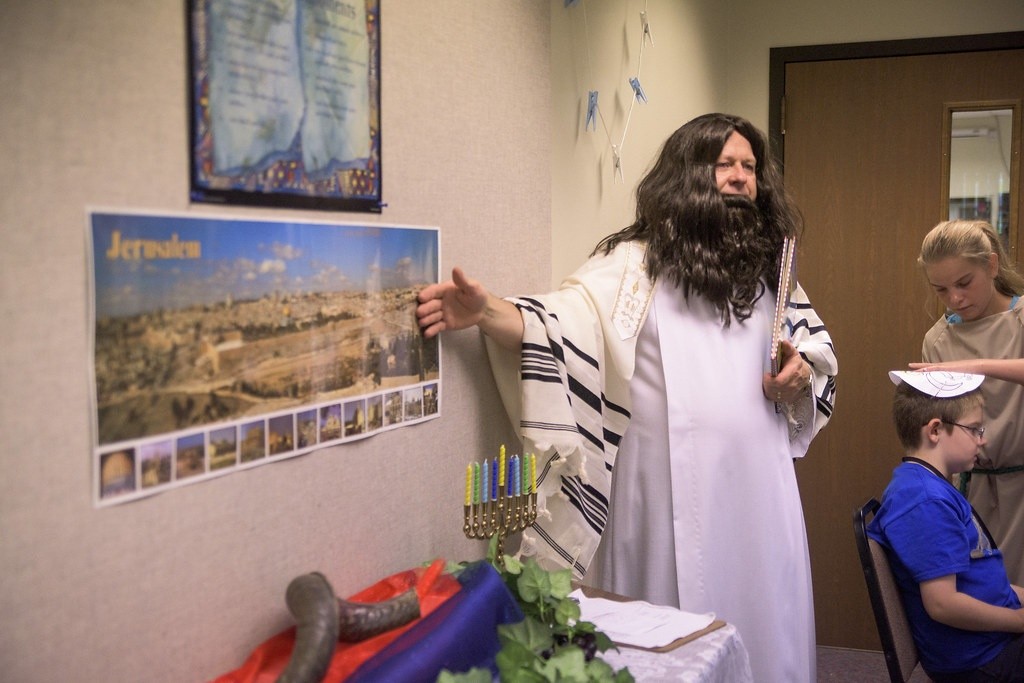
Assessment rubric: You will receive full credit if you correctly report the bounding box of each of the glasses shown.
[940,419,985,441]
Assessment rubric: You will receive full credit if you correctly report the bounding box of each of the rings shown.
[777,393,781,401]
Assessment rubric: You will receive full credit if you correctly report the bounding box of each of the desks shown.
[211,559,758,683]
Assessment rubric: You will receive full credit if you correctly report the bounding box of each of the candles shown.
[482,458,488,502]
[522,452,529,492]
[490,456,498,499]
[507,455,514,495]
[514,454,520,493]
[498,445,505,487]
[464,461,472,505]
[473,461,480,504]
[531,453,537,491]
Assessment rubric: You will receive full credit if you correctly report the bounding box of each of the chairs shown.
[856,497,936,683]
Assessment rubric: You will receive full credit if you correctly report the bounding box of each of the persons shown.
[908,218,1024,587]
[865,371,1024,683]
[417,113,839,683]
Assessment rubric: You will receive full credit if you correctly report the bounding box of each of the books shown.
[770,235,797,413]
